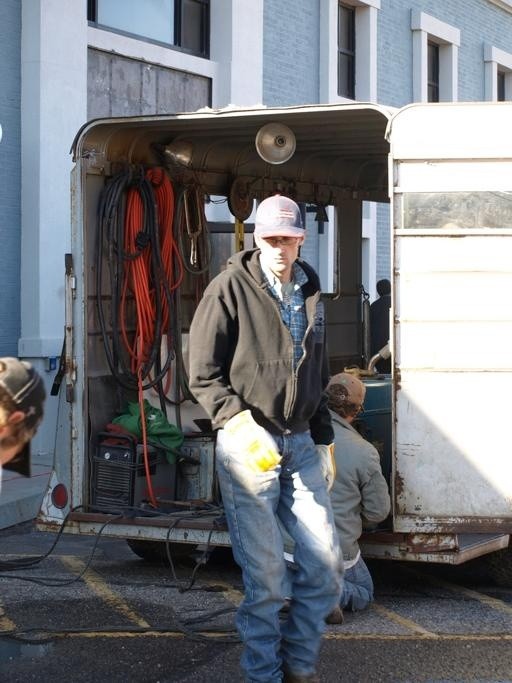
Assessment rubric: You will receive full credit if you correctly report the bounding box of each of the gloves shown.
[222,409,283,472]
[314,441,336,492]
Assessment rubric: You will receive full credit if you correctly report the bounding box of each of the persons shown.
[370,278,391,374]
[276,372,391,625]
[0,356,46,467]
[188,193,345,683]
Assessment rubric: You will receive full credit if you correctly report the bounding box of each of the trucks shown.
[33,93,512,590]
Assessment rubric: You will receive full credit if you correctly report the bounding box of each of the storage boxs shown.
[354,371,392,476]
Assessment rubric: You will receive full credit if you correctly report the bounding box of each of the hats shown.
[252,193,306,239]
[325,373,367,405]
[1,358,48,479]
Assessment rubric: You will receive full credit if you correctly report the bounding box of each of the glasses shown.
[261,238,298,245]
